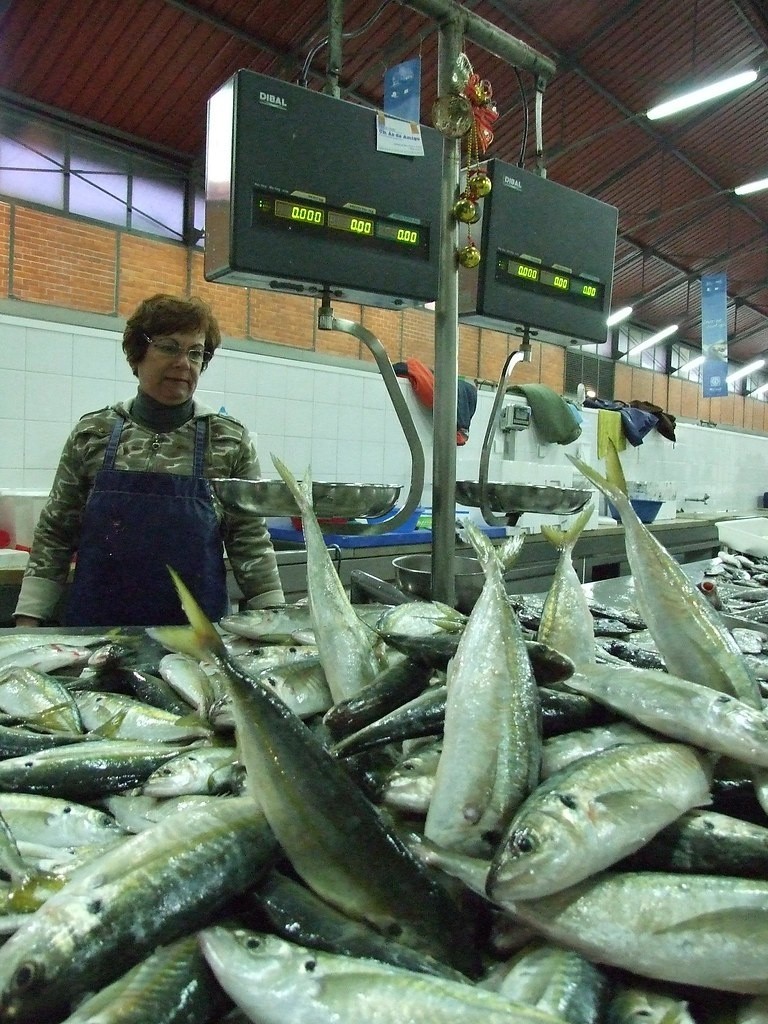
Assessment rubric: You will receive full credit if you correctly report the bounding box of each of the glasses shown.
[143,332,211,363]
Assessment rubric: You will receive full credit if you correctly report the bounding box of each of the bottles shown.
[626,481,676,521]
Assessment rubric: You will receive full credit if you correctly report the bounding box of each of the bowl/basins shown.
[715,517,768,558]
[608,498,665,524]
[367,506,425,533]
[392,555,486,613]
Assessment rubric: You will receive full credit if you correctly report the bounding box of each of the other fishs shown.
[1,434,768,1024]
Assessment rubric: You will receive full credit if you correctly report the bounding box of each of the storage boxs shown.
[715,512,768,559]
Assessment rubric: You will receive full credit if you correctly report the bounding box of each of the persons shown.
[11,293,285,629]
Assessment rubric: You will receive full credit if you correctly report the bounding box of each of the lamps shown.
[749,382,768,398]
[679,355,706,374]
[628,323,679,357]
[606,303,634,330]
[725,354,765,385]
[645,65,760,119]
[735,176,768,195]
